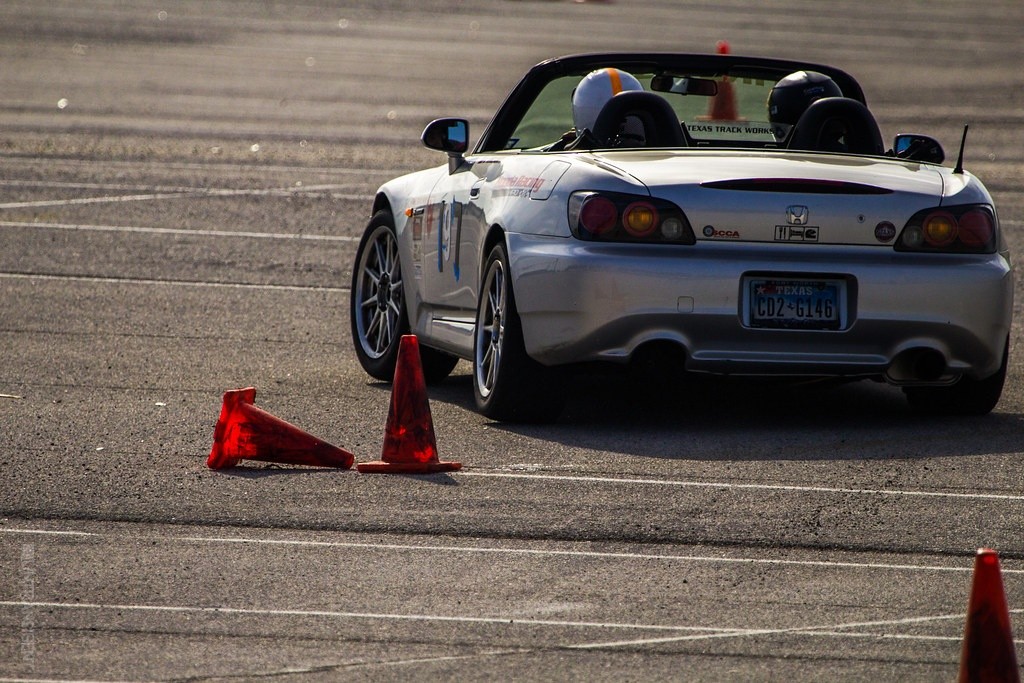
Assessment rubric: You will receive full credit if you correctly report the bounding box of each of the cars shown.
[349,50,1013,422]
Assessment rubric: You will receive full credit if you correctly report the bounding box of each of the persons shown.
[767,70,845,149]
[561,68,646,150]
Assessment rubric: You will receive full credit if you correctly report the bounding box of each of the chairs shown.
[591,90,689,147]
[785,96,884,154]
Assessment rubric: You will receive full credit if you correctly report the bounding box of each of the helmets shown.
[574,68,644,135]
[766,70,843,142]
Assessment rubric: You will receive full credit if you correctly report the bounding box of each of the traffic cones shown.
[959,548,1020,683]
[356,334,462,475]
[207,386,355,470]
[695,42,747,125]
